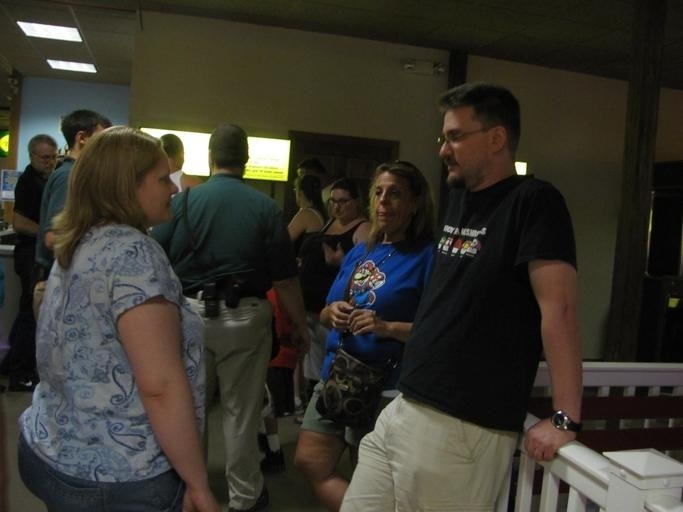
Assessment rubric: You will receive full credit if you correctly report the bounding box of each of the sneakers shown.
[257,432,285,473]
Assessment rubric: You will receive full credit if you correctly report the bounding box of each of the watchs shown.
[547,410,582,432]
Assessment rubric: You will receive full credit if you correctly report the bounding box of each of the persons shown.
[269,159,372,423]
[15,126,220,511]
[338,81,582,511]
[152,125,311,511]
[7,110,112,390]
[294,160,438,511]
[160,134,202,197]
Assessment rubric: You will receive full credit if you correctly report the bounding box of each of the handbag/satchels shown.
[314,349,386,433]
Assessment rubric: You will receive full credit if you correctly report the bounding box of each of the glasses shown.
[437,128,489,145]
[328,198,352,207]
